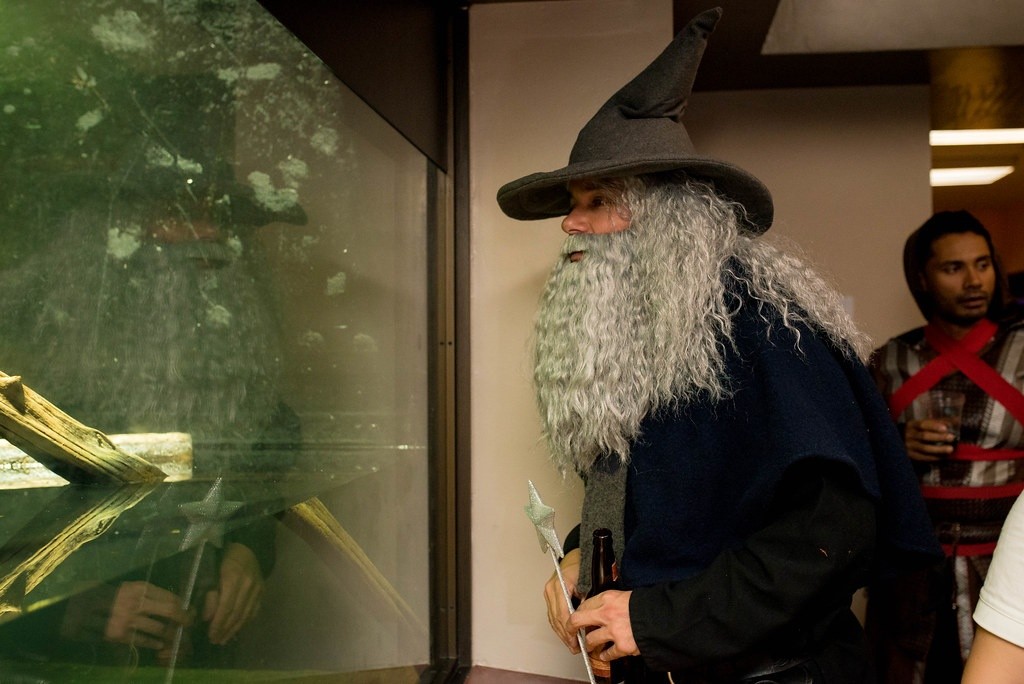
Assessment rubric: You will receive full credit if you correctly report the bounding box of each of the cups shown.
[922,391,963,456]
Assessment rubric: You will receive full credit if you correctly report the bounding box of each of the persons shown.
[495,3,1024,683]
[1,70,306,666]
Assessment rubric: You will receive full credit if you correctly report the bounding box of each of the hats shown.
[33,73,303,227]
[496,5,773,239]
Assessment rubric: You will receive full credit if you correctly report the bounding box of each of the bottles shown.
[585,529,638,684]
